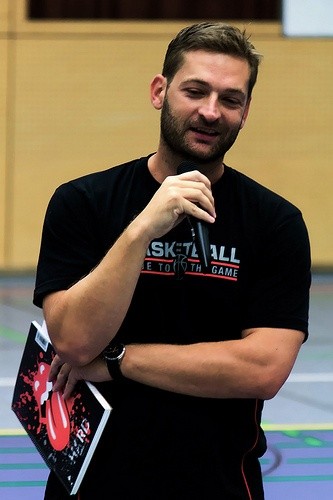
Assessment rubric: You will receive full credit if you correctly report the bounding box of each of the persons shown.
[32,22,312,500]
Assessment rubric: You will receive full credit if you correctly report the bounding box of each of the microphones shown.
[176,161,211,268]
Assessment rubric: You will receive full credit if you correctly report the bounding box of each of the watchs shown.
[101,341,131,380]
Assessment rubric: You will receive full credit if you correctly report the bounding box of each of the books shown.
[12,320,113,495]
